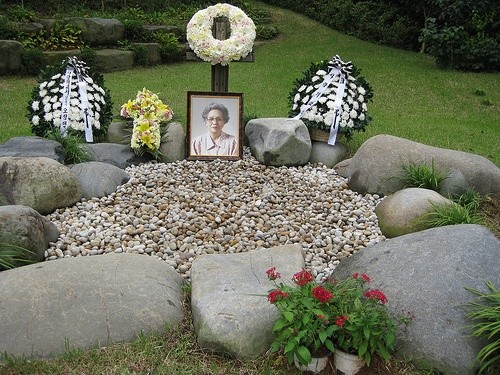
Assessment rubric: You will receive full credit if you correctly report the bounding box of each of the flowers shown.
[119,87,175,156]
[287,51,372,145]
[264,260,350,364]
[187,2,258,68]
[27,56,109,143]
[325,269,416,366]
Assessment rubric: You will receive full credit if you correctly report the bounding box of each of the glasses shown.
[207,117,221,121]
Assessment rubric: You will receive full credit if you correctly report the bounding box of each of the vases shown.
[292,347,333,373]
[333,345,365,375]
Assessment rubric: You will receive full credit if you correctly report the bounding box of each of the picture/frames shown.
[186,90,243,161]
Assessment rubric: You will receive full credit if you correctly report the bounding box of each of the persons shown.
[190,102,239,156]
